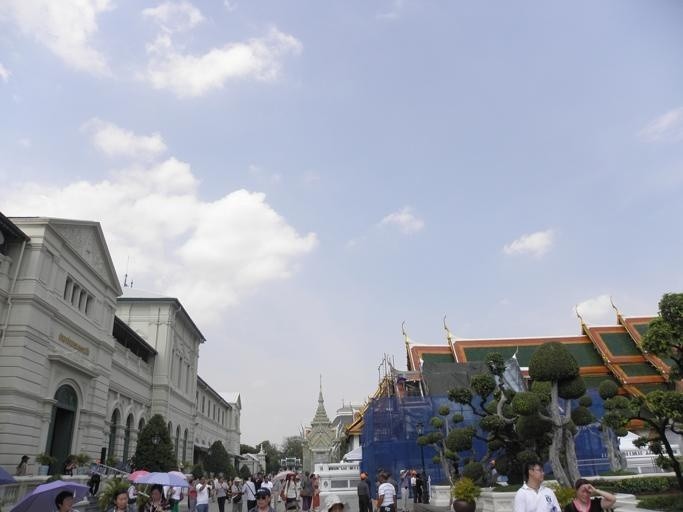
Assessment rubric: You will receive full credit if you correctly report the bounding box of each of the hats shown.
[254,489,270,496]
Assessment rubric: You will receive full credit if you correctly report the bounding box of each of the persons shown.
[513,460,561,511]
[108,484,183,512]
[489,459,502,483]
[564,477,615,511]
[400,469,428,511]
[242,467,320,511]
[372,469,398,511]
[188,472,243,511]
[65,460,101,497]
[358,471,373,511]
[16,455,30,475]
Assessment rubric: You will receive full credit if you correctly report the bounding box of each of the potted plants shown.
[450,477,481,511]
[417,404,495,508]
[34,452,90,476]
[449,342,597,511]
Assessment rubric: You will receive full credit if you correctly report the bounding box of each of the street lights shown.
[414,419,429,504]
[149,429,161,450]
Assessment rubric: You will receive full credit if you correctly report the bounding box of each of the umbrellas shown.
[9,480,90,511]
[0,466,16,484]
